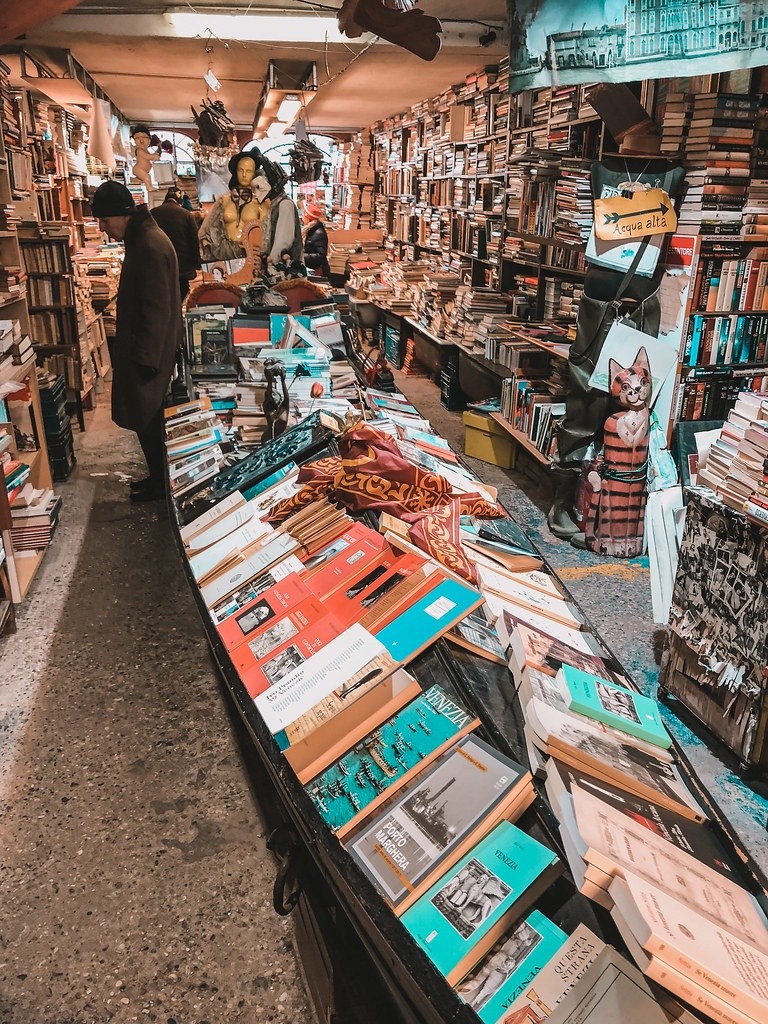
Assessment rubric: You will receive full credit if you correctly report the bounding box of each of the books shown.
[319,51,768,296]
[143,173,203,212]
[0,77,146,650]
[167,296,768,1023]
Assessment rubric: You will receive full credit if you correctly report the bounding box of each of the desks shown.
[656,483,768,780]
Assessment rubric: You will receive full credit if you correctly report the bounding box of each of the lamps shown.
[267,119,289,138]
[277,94,301,122]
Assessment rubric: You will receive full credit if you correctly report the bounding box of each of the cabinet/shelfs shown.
[0,44,768,636]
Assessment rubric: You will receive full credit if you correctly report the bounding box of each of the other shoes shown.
[130,477,150,489]
[130,489,166,502]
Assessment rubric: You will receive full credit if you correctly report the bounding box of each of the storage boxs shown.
[47,424,77,483]
[439,369,471,411]
[385,325,401,369]
[462,410,519,469]
[446,345,460,378]
[344,284,367,299]
[39,374,69,433]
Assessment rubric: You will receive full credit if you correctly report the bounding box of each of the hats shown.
[303,205,322,221]
[92,180,138,217]
[602,134,681,159]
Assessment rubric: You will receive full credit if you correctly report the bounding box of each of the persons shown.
[278,250,302,279]
[90,180,185,503]
[300,204,331,284]
[148,185,203,302]
[130,124,162,191]
[248,267,266,288]
[198,155,271,262]
[259,252,286,285]
[265,187,303,276]
